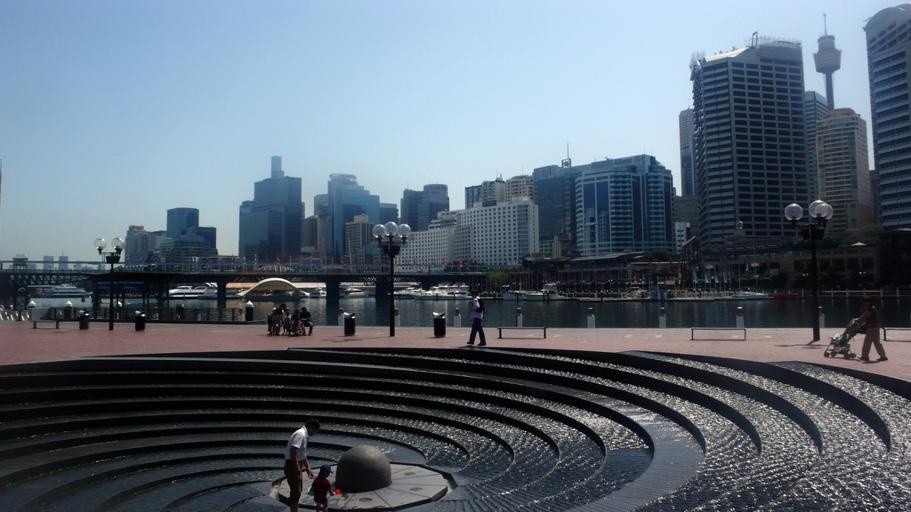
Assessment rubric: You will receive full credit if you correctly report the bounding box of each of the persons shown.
[856,294,886,363]
[313,465,334,509]
[270,302,313,336]
[467,291,487,346]
[284,418,313,508]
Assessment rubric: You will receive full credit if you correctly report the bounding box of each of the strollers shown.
[824,316,861,360]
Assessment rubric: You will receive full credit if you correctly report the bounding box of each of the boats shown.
[731,289,769,301]
[25,283,123,311]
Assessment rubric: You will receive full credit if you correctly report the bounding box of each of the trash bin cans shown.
[344,312,356,336]
[79,310,89,329]
[135,311,146,331]
[433,312,446,338]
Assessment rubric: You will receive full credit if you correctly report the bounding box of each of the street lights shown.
[372,220,413,337]
[95,236,124,330]
[785,199,834,343]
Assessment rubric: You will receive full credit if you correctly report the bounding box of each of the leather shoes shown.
[856,356,867,361]
[876,358,886,361]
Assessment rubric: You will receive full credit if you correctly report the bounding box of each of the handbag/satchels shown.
[474,299,484,312]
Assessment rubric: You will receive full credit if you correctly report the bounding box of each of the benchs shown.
[497,327,547,339]
[691,327,746,341]
[883,327,911,340]
[33,320,59,329]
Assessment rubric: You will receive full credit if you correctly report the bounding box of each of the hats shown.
[319,465,333,478]
